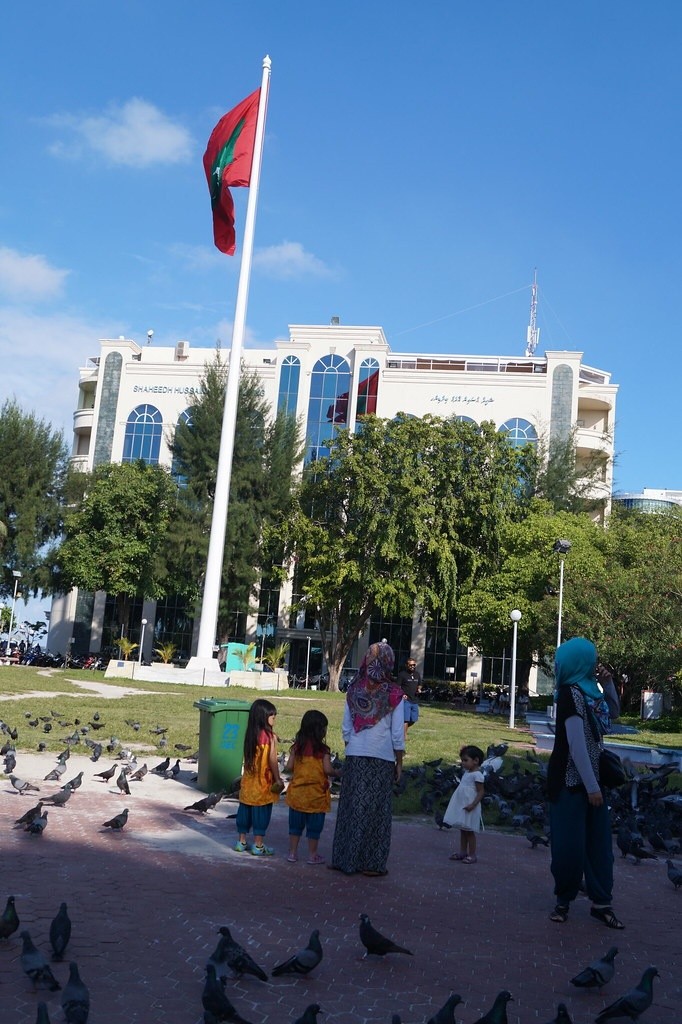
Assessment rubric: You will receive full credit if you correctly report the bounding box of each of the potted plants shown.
[104,637,140,678]
[152,640,180,668]
[228,641,289,690]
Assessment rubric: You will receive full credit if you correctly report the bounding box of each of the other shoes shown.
[288,851,299,862]
[307,855,325,865]
[450,852,478,864]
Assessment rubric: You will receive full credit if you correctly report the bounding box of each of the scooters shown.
[0,647,108,673]
[296,674,529,716]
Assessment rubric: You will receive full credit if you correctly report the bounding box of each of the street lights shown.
[509,609,521,728]
[6,571,21,657]
[139,619,148,662]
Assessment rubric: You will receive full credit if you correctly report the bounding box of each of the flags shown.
[327,369,378,422]
[203,87,261,255]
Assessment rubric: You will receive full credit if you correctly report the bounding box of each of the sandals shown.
[591,905,625,930]
[551,904,570,923]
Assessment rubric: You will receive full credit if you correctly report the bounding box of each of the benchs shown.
[0,657,20,665]
[451,687,467,710]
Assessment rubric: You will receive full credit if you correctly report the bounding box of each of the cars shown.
[313,668,360,689]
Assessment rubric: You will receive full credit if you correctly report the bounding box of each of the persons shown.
[288,710,343,864]
[396,658,422,742]
[545,637,627,929]
[442,745,485,864]
[235,700,286,856]
[326,641,406,876]
[0,639,102,671]
[451,684,531,717]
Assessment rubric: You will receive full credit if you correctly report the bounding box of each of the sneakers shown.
[234,840,250,851]
[251,844,275,856]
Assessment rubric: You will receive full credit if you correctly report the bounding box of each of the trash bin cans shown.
[192,698,251,794]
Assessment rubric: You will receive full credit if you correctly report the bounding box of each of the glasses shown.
[409,664,416,667]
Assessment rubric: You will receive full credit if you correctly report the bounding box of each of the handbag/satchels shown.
[601,747,625,789]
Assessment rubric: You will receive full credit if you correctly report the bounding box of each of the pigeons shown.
[0,710,225,839]
[293,1004,324,1024]
[594,967,661,1024]
[568,948,620,996]
[215,927,268,983]
[0,895,20,944]
[48,901,72,961]
[35,1001,51,1024]
[272,929,323,980]
[60,960,89,1024]
[204,963,252,1024]
[357,914,416,962]
[392,989,572,1024]
[222,729,681,892]
[18,930,62,994]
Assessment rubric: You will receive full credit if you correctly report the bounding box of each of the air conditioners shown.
[175,340,191,359]
